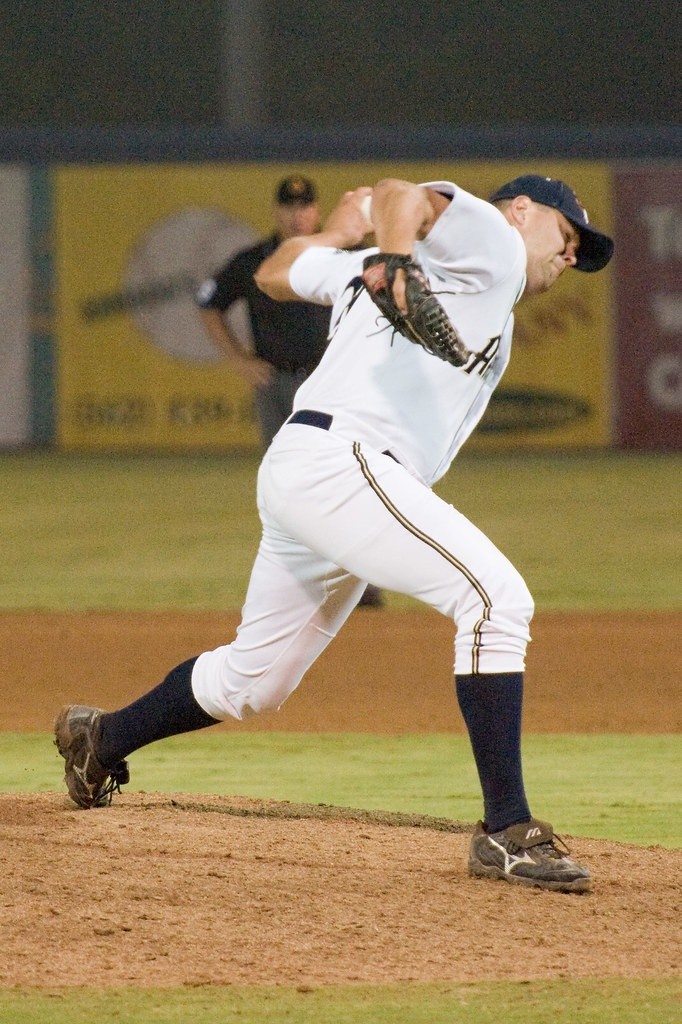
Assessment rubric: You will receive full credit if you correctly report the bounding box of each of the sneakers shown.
[468,817,595,893]
[54,705,130,808]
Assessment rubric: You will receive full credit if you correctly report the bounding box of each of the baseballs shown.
[361,195,373,222]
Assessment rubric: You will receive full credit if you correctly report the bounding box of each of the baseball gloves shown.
[362,253,469,367]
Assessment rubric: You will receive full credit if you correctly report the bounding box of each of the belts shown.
[287,410,399,462]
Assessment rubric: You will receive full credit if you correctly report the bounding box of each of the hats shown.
[278,174,315,205]
[488,175,615,272]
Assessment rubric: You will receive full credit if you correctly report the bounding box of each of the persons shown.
[193,174,334,452]
[52,173,614,892]
